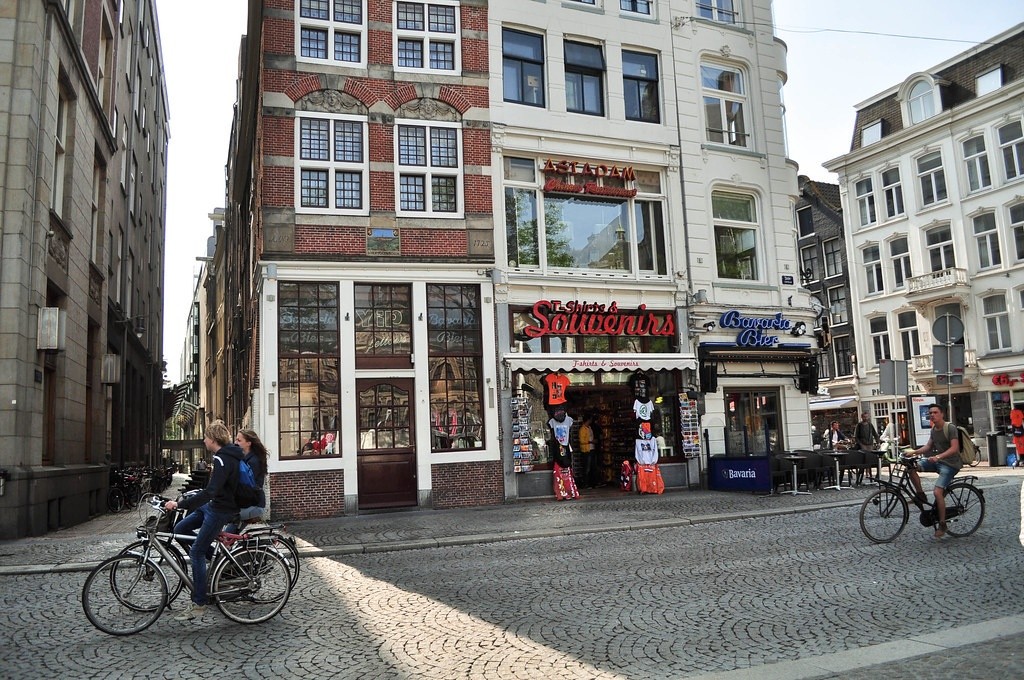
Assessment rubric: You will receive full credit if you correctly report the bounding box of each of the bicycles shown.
[80,464,301,638]
[858,451,987,546]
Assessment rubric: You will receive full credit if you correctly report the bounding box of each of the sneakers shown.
[174,601,208,620]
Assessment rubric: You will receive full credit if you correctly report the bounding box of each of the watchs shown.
[935,454,940,460]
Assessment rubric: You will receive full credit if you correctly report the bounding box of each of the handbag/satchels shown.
[239,459,257,488]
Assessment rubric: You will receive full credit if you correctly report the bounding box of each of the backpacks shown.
[943,422,979,464]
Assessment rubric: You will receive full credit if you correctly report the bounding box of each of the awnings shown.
[808,394,859,410]
[500,353,699,391]
[165,380,199,430]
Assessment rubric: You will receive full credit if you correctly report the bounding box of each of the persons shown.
[824,420,851,452]
[855,412,882,479]
[196,457,207,471]
[165,420,246,620]
[578,414,608,489]
[905,403,963,539]
[224,428,271,546]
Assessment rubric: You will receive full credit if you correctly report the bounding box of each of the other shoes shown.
[934,522,949,537]
[908,491,927,505]
